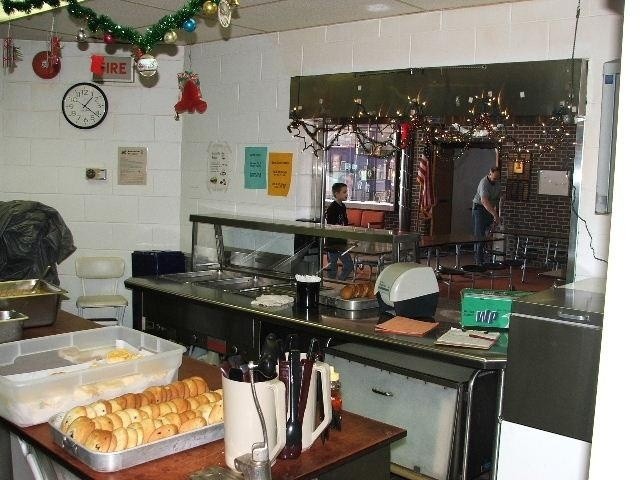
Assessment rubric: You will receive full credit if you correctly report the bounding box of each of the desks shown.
[0,309,410,478]
[324,227,507,294]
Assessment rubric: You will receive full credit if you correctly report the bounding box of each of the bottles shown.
[329,365,343,433]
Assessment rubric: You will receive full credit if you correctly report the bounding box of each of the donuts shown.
[33,52,60,79]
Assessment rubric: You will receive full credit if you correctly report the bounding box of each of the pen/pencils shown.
[469,333,496,343]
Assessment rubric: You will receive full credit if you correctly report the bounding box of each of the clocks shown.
[63,82,107,131]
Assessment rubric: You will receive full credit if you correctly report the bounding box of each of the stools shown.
[431,250,539,304]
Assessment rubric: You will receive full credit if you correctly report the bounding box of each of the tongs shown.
[278,333,330,460]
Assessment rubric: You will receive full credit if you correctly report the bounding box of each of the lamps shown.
[287,75,571,174]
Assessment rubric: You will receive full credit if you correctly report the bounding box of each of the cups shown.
[223,353,329,463]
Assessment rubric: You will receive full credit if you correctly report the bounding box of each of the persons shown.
[471,167,504,265]
[325,182,354,281]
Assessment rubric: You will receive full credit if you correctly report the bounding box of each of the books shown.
[373,316,440,338]
[433,327,501,349]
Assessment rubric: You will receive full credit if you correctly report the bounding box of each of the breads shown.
[59,375,225,452]
[341,282,376,299]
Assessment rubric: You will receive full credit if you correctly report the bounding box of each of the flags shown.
[416,139,438,220]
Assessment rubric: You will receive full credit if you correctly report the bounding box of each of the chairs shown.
[71,254,129,324]
